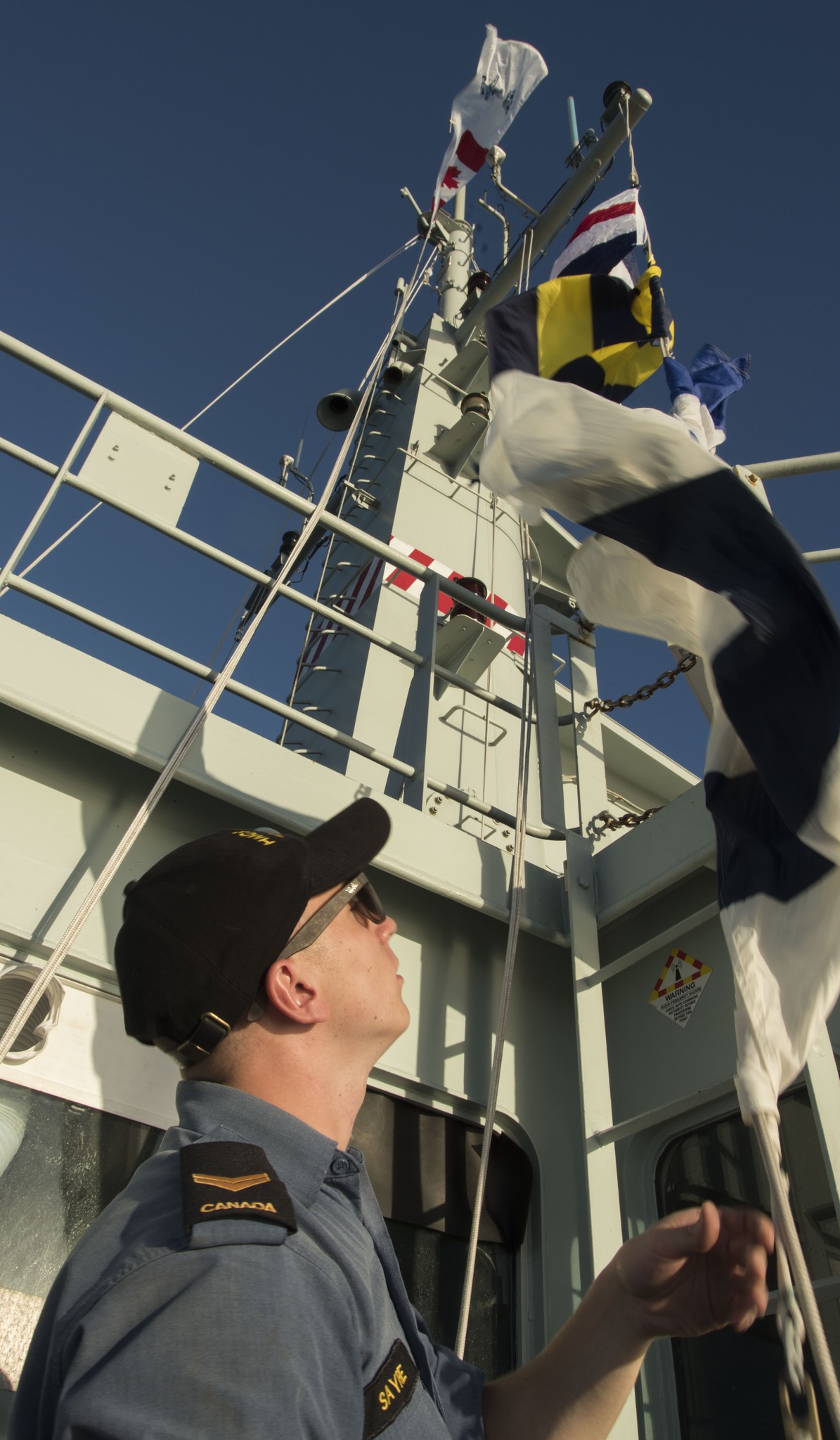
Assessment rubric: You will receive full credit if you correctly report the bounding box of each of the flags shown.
[428,22,840,1123]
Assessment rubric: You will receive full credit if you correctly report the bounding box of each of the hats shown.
[112,796,392,1068]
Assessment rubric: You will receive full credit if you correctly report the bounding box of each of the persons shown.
[11,798,775,1440]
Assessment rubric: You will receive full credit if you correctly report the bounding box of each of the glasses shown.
[247,868,386,1022]
